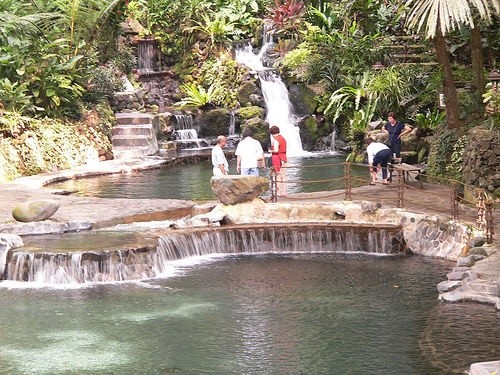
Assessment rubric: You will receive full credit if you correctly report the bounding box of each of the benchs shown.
[388,162,424,188]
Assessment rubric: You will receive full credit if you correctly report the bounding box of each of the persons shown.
[382,112,411,161]
[267,126,287,174]
[365,138,393,185]
[211,135,229,176]
[235,127,266,175]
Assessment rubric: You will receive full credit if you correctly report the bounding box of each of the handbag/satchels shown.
[386,142,392,149]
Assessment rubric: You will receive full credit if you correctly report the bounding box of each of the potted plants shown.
[415,109,447,137]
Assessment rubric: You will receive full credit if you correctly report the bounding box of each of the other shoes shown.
[383,183,389,185]
[369,183,376,186]
